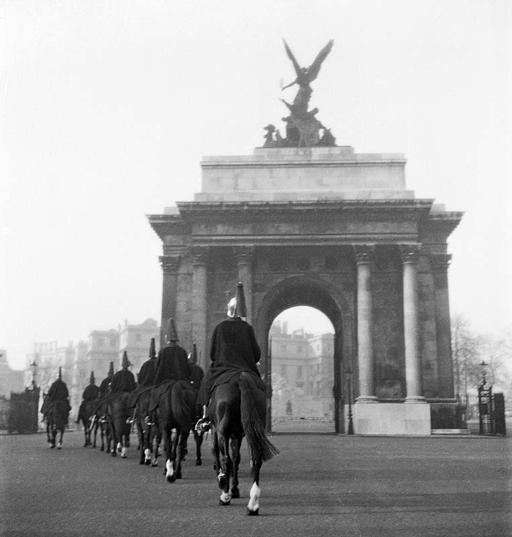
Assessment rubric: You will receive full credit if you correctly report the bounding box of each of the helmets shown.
[227,297,242,318]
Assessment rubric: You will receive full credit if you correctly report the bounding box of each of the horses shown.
[42,392,67,449]
[80,380,204,483]
[206,371,281,516]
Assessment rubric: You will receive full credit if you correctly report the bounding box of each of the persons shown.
[25,317,205,428]
[285,399,292,415]
[194,281,261,436]
[274,33,334,115]
